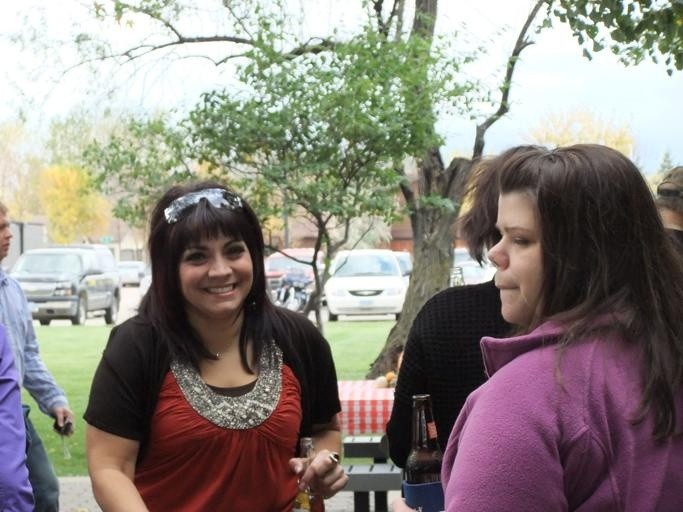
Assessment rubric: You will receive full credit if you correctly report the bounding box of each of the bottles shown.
[402,394,445,512]
[290,437,326,512]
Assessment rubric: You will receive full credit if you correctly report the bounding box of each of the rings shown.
[329,450,340,467]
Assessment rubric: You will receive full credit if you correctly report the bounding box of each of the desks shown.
[338,380,395,437]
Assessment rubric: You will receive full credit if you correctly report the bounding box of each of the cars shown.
[264,247,327,310]
[119,260,144,286]
[324,248,407,321]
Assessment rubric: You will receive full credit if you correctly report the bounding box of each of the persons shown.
[80,179,350,512]
[655,165,682,262]
[385,142,548,470]
[0,202,76,511]
[390,141,682,510]
[0,323,37,511]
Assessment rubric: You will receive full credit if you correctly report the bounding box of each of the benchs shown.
[342,465,404,512]
[344,436,387,463]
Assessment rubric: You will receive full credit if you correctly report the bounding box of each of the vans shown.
[9,242,123,326]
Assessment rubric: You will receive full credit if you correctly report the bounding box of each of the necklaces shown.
[203,328,240,359]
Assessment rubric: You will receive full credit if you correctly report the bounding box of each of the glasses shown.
[159,187,245,224]
